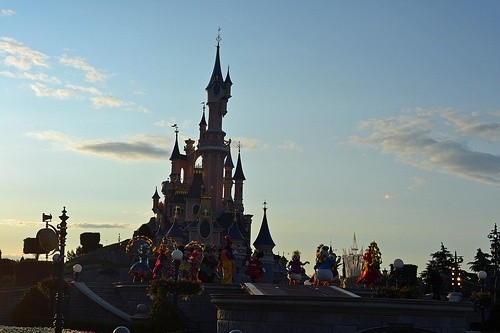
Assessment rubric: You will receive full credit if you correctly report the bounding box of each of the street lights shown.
[477,270,489,333]
[36,206,70,333]
[170,249,183,307]
[393,258,404,294]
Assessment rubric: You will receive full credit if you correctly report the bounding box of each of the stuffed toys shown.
[312,246,341,288]
[287,252,309,288]
[244,250,265,283]
[125,241,237,284]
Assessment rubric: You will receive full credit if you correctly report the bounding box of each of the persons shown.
[428,267,443,300]
[355,252,382,290]
